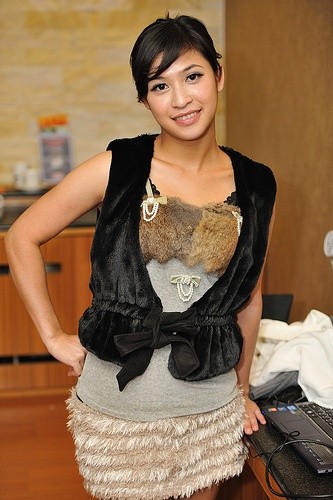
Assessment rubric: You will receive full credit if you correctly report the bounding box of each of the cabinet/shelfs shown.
[0,193,114,364]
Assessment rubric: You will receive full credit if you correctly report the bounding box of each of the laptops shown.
[263,403,333,473]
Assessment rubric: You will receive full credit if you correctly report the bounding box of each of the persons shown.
[3,11,277,500]
